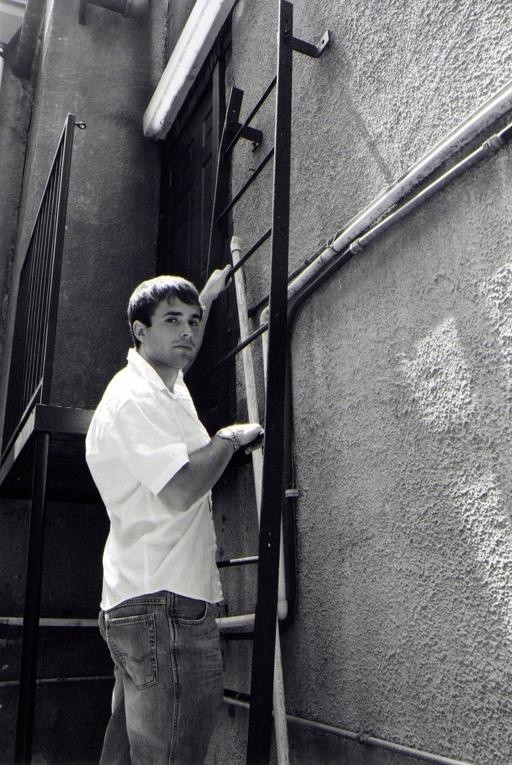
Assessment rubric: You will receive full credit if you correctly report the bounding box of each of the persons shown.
[86,264,264,764]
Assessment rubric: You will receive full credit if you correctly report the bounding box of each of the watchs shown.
[216,428,241,452]
